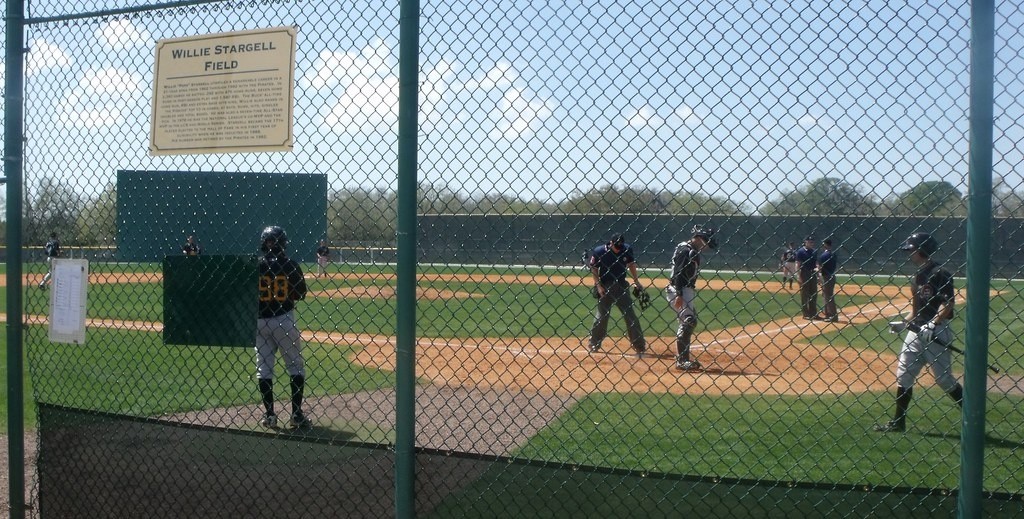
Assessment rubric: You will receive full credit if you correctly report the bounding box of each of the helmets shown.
[260,226,289,252]
[902,232,938,256]
[691,223,716,248]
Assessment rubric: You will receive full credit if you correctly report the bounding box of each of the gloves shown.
[886,320,907,334]
[918,321,936,343]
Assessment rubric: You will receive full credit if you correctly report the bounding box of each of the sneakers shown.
[874,419,905,432]
[261,412,277,428]
[675,359,700,372]
[289,413,314,427]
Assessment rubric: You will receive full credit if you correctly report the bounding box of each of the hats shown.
[822,238,832,243]
[611,233,624,250]
[804,235,815,240]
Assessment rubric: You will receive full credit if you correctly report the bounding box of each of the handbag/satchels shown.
[590,284,599,298]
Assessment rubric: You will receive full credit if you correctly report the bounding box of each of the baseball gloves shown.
[632,286,650,303]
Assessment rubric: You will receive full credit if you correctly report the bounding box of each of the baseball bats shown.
[905,321,1000,374]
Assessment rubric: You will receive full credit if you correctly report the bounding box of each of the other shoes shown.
[629,339,647,353]
[38,285,46,292]
[804,313,822,320]
[781,284,784,289]
[824,314,838,323]
[587,338,602,351]
[789,286,793,290]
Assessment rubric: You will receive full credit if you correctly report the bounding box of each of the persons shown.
[182,236,201,255]
[581,248,588,272]
[795,235,822,320]
[666,226,717,369]
[817,239,838,322]
[781,241,798,290]
[873,232,963,431]
[38,232,60,290]
[316,239,329,277]
[588,232,646,353]
[255,225,312,427]
